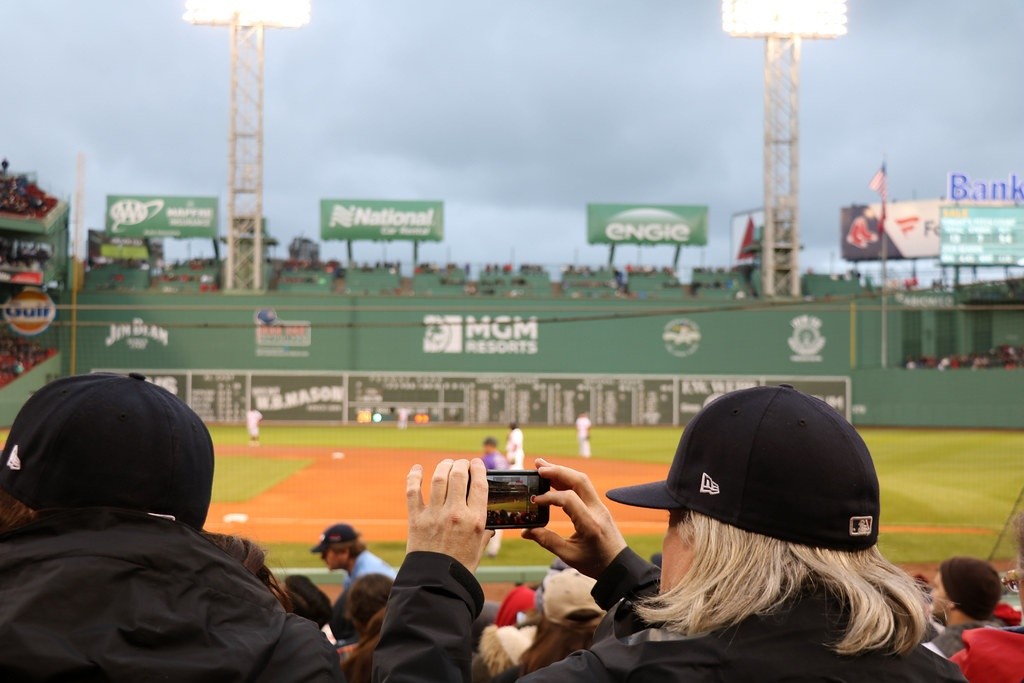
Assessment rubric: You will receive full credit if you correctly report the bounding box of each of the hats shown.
[605,384,880,550]
[545,568,607,630]
[940,558,1002,624]
[313,525,357,553]
[496,584,539,629]
[0,373,215,533]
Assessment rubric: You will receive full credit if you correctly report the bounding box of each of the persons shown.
[626,263,679,288]
[690,267,741,288]
[421,263,470,285]
[480,437,506,557]
[334,574,394,683]
[471,512,1024,683]
[0,174,48,218]
[567,264,623,295]
[0,373,345,683]
[506,422,524,471]
[0,334,41,375]
[0,237,49,267]
[311,524,398,610]
[576,412,591,458]
[371,383,969,683]
[486,263,541,285]
[90,257,402,292]
[905,345,1024,371]
[245,408,263,446]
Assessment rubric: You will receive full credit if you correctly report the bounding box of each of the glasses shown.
[1002,569,1024,593]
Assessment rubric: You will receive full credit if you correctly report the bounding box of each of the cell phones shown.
[466,471,551,529]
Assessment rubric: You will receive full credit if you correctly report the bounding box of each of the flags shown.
[869,166,887,201]
